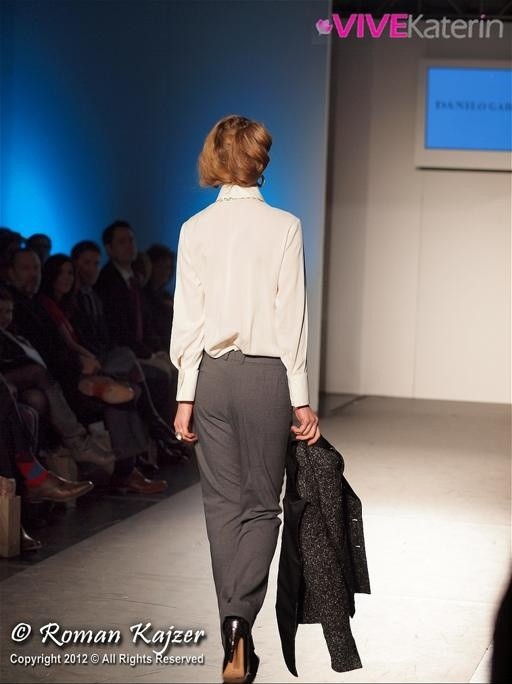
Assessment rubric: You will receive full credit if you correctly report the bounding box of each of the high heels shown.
[221,617,260,683]
[149,416,184,449]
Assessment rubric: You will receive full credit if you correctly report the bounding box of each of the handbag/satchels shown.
[0,475,22,560]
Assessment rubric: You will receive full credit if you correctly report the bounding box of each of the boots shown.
[45,380,126,463]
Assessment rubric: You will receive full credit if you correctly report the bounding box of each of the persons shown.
[168,112,322,684]
[0,214,199,568]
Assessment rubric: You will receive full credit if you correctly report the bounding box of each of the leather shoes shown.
[19,523,43,550]
[116,467,170,493]
[21,470,95,503]
[160,438,192,460]
[77,372,134,403]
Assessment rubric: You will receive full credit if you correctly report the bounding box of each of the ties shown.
[87,294,99,329]
[128,276,144,342]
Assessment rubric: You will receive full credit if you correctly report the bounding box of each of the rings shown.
[174,431,183,442]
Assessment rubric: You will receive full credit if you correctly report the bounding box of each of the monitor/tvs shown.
[410,56,512,173]
